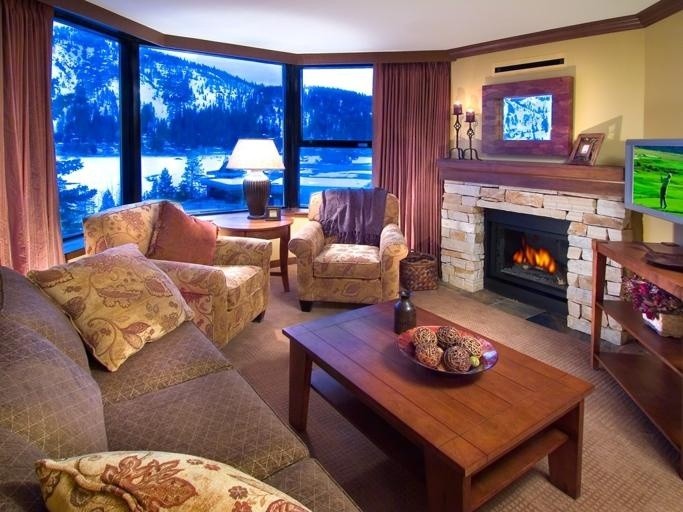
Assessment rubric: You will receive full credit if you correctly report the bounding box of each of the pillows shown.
[32,450,313,512]
[144,200,220,269]
[23,240,195,374]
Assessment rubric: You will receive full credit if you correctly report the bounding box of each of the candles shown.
[453,102,462,114]
[465,109,475,122]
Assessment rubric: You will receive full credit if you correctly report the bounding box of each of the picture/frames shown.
[564,131,605,168]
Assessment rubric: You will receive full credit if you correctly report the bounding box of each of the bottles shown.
[393,289,416,335]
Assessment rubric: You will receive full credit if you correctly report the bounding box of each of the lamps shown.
[223,135,287,219]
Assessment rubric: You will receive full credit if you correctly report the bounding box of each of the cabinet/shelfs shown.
[586,236,682,470]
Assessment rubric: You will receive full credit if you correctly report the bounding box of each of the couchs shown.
[284,188,409,313]
[0,267,363,512]
[77,201,275,357]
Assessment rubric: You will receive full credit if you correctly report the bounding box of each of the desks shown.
[209,213,295,294]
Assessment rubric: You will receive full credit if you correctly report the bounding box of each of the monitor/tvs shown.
[624,139,683,225]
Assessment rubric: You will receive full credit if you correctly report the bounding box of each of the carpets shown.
[217,262,681,512]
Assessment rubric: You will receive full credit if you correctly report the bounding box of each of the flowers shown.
[618,272,682,321]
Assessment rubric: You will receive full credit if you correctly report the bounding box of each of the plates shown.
[397,325,498,376]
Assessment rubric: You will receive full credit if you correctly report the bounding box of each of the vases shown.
[640,308,682,340]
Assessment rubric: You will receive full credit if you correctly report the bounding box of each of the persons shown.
[659,173,672,209]
